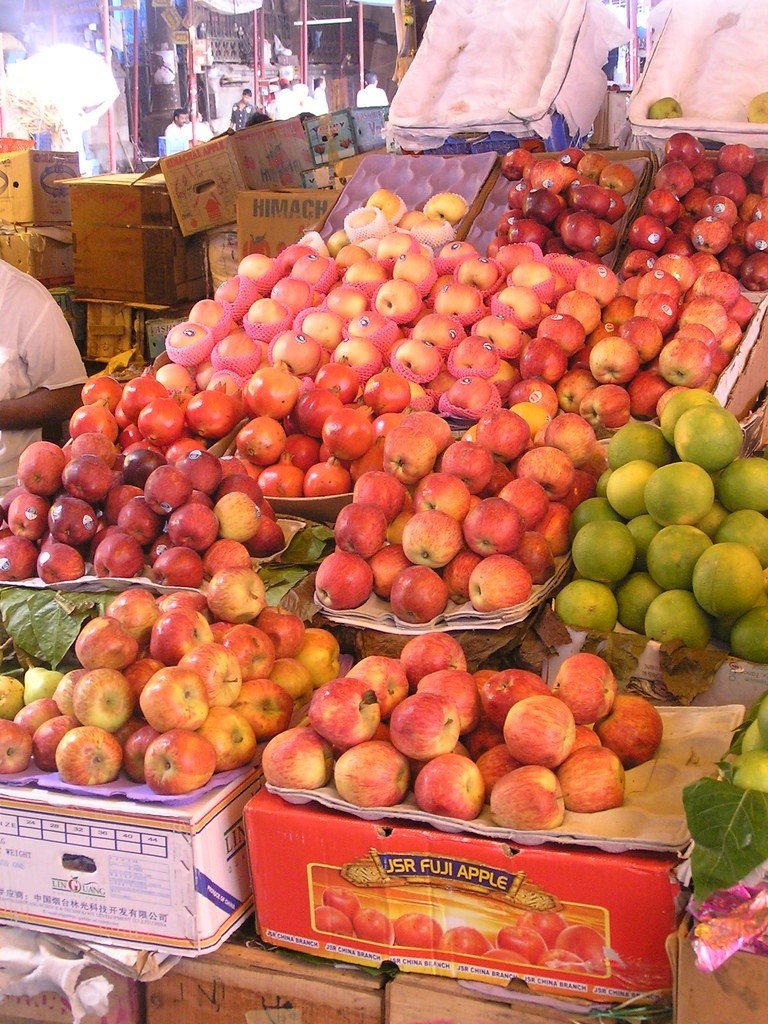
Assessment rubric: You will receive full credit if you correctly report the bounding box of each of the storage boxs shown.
[87,304,145,360]
[144,936,385,1024]
[303,106,391,167]
[69,184,213,307]
[0,151,80,226]
[314,0,768,272]
[146,317,188,359]
[301,165,337,190]
[0,764,262,959]
[245,705,768,1024]
[236,189,342,265]
[0,226,73,289]
[133,117,315,237]
[0,928,144,1024]
[714,292,768,455]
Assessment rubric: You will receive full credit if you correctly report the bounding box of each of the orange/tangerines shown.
[555,386,768,664]
[747,92,768,124]
[734,695,768,794]
[649,97,682,118]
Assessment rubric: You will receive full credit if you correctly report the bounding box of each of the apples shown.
[261,634,662,829]
[2,133,768,621]
[315,889,607,974]
[243,233,271,258]
[1,616,338,795]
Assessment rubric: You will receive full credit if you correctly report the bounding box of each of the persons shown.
[166,109,213,157]
[1,259,89,496]
[356,73,388,108]
[313,77,329,115]
[229,89,255,131]
